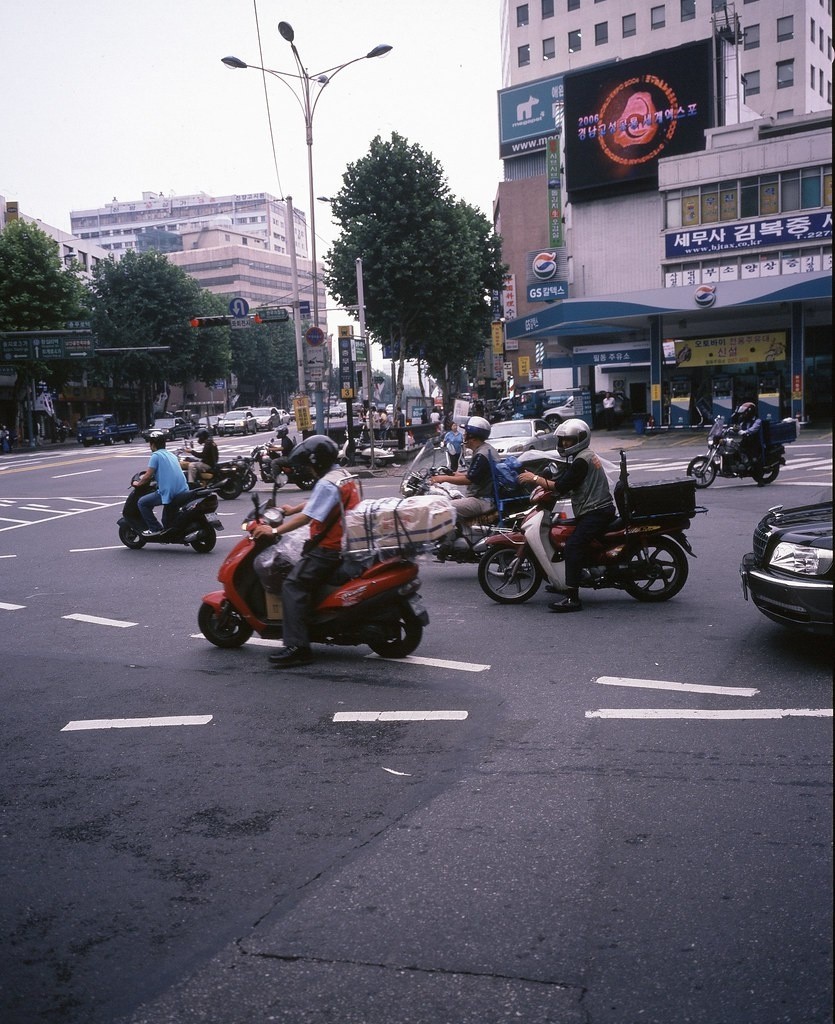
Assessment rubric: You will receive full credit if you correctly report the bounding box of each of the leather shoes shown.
[547,597,583,611]
[545,585,569,593]
[266,645,314,665]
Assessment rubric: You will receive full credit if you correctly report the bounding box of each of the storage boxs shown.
[347,495,456,548]
[265,590,285,621]
[626,477,697,522]
[767,419,798,446]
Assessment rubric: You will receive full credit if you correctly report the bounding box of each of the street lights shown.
[221,22,393,434]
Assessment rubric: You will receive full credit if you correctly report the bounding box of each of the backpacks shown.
[481,451,528,498]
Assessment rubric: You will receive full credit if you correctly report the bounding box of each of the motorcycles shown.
[686,415,797,489]
[398,440,531,563]
[477,452,708,604]
[54,425,72,443]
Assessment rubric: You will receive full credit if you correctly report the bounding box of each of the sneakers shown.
[141,529,160,537]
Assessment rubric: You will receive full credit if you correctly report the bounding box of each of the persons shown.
[395,407,406,428]
[0,426,7,455]
[358,405,389,441]
[513,419,616,613]
[184,427,218,482]
[730,402,765,471]
[265,424,293,479]
[430,408,440,422]
[132,430,188,537]
[425,416,503,519]
[442,421,464,472]
[602,392,617,431]
[252,434,359,665]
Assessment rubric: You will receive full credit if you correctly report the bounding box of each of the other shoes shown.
[757,478,764,487]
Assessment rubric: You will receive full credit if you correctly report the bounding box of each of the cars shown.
[491,420,558,461]
[739,500,832,637]
[141,400,395,443]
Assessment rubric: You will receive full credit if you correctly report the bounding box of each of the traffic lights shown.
[191,316,232,329]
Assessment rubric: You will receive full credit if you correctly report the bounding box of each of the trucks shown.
[79,414,139,447]
[490,389,590,431]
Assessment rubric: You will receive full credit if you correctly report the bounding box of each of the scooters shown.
[198,472,430,657]
[116,471,224,553]
[116,437,394,500]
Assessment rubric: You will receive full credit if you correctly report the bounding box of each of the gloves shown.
[738,429,746,435]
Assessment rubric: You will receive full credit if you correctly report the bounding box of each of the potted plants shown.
[58,429,68,443]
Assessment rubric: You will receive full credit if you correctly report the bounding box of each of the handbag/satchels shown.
[301,535,322,556]
[460,454,465,467]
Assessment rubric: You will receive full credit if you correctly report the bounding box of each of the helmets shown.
[148,430,165,443]
[195,429,208,444]
[275,425,290,438]
[460,417,491,441]
[737,403,756,421]
[553,418,591,457]
[288,434,339,477]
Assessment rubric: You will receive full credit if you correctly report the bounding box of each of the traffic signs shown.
[0,334,96,365]
